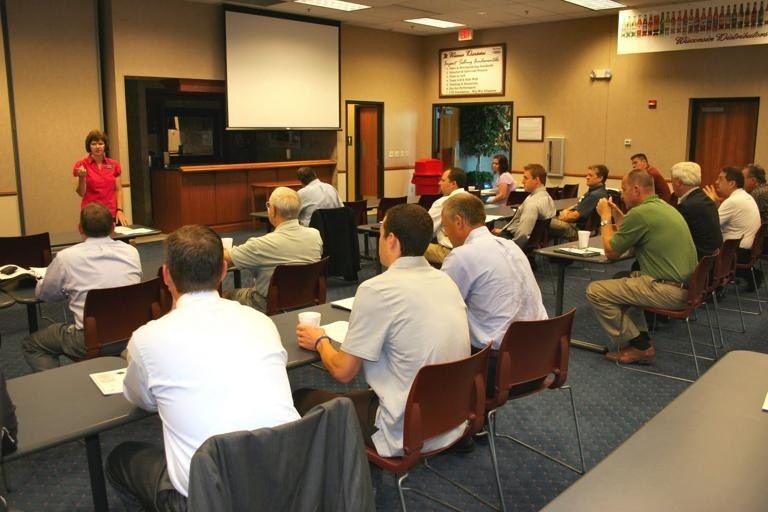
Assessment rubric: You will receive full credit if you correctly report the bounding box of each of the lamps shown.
[590,69,612,78]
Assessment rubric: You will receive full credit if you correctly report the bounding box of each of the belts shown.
[657,279,689,289]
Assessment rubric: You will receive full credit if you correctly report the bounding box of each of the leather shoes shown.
[605,335,656,365]
[745,270,763,291]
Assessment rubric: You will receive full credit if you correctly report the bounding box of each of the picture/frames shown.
[438,42,506,98]
[516,115,545,142]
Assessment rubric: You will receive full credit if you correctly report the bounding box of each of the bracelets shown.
[116,208,124,212]
[315,336,331,350]
[600,220,612,226]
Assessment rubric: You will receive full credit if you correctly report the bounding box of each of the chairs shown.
[1,186,766,512]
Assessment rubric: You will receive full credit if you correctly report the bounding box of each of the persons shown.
[630,154,671,203]
[440,192,550,442]
[704,166,764,293]
[1,374,18,512]
[428,167,467,263]
[105,222,301,511]
[586,168,699,363]
[296,166,345,227]
[488,154,515,207]
[293,202,469,458]
[23,203,143,373]
[549,165,609,239]
[493,164,556,251]
[743,164,768,220]
[72,131,129,245]
[669,162,723,284]
[223,186,324,316]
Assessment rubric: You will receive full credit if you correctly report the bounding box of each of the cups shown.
[578,231,591,249]
[297,311,321,327]
[221,237,233,249]
[468,186,476,192]
